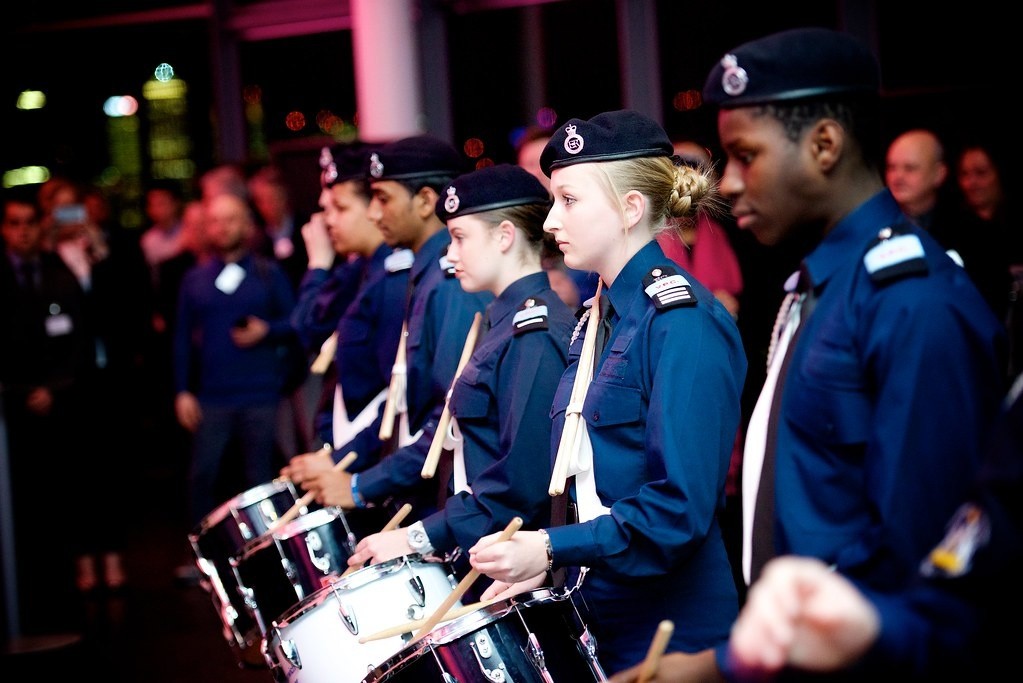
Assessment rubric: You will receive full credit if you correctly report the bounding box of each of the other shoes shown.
[172,565,198,581]
[76,556,97,590]
[104,553,126,588]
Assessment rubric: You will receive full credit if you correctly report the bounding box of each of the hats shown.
[318,140,382,188]
[435,164,550,225]
[540,110,674,179]
[703,29,881,108]
[363,136,467,179]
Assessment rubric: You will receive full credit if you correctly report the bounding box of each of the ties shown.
[383,281,414,462]
[750,284,819,582]
[304,260,365,452]
[438,314,490,514]
[21,262,35,291]
[552,295,613,589]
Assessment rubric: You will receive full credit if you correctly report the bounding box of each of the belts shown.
[564,503,579,526]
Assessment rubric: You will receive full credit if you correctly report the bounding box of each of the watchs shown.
[407,519,436,556]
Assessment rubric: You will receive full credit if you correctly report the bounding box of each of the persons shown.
[604,30,982,683]
[511,124,1023,562]
[731,360,1023,683]
[279,136,492,508]
[0,178,124,588]
[137,163,294,583]
[347,108,740,676]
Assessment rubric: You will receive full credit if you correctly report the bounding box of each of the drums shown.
[266,550,473,683]
[359,584,613,683]
[222,504,367,672]
[184,473,312,651]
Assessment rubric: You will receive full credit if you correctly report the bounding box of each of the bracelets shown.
[350,473,365,507]
[538,529,553,573]
